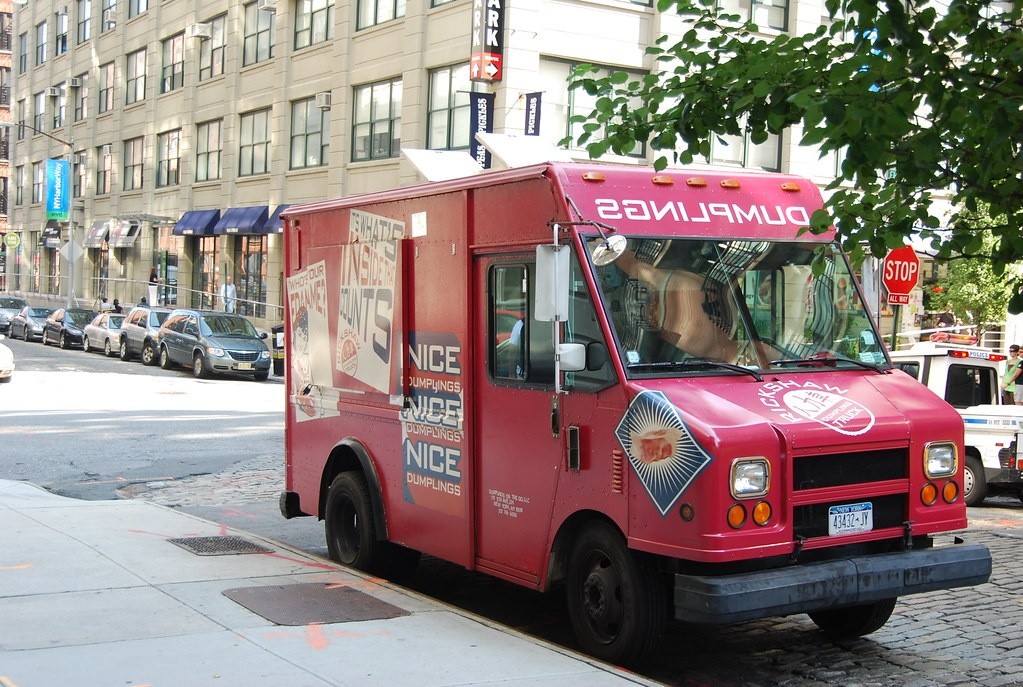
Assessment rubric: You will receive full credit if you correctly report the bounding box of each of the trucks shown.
[279,134,992,664]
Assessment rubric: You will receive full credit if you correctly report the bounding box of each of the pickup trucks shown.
[880,332,1023,507]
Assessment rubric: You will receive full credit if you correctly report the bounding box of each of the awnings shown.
[172,209,220,236]
[39,219,61,246]
[83,220,110,249]
[213,206,269,234]
[109,224,142,248]
[263,203,293,234]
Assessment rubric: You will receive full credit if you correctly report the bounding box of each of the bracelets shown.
[1007,383,1010,385]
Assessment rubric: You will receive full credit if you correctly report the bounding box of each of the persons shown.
[138,297,149,306]
[938,302,964,326]
[221,275,236,312]
[149,268,161,306]
[101,298,112,309]
[112,299,124,314]
[508,318,524,378]
[1003,346,1023,405]
[1001,345,1021,404]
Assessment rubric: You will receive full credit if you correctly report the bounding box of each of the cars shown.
[8,306,53,341]
[43,308,98,349]
[82,313,127,357]
[0,334,15,383]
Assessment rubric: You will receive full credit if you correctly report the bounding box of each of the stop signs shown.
[881,244,919,294]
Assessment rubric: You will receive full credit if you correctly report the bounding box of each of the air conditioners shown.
[59,5,68,15]
[103,145,112,156]
[45,87,60,96]
[68,78,82,87]
[190,23,211,36]
[315,92,331,108]
[104,10,117,23]
[67,153,81,164]
[258,0,276,11]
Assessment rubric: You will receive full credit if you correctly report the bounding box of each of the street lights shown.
[0,122,75,308]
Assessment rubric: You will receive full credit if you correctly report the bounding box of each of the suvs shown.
[119,305,175,366]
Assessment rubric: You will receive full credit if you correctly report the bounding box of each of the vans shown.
[0,296,31,333]
[158,309,271,381]
